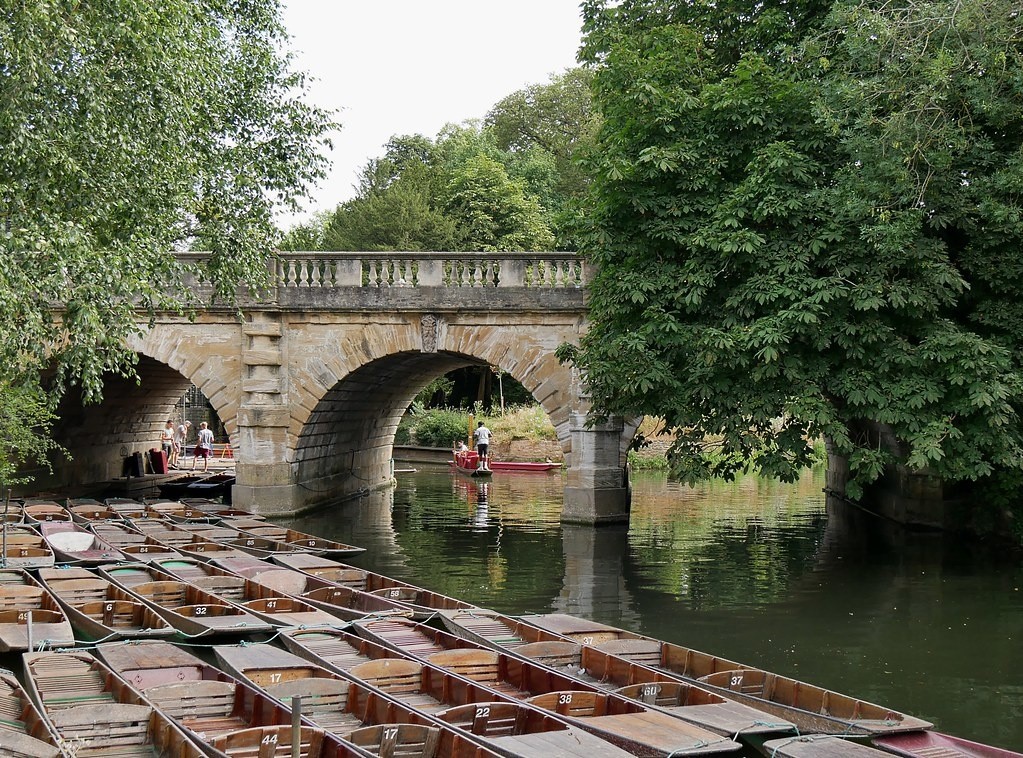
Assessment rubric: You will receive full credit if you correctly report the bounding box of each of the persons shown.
[453,440,469,456]
[190,421,214,473]
[170,420,192,469]
[161,419,176,468]
[473,421,493,471]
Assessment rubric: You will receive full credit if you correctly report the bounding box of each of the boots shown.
[484,461,488,470]
[479,461,483,470]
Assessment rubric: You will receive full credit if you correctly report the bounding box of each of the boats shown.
[447,440,565,472]
[187,470,236,502]
[156,474,208,497]
[1,492,1022,757]
